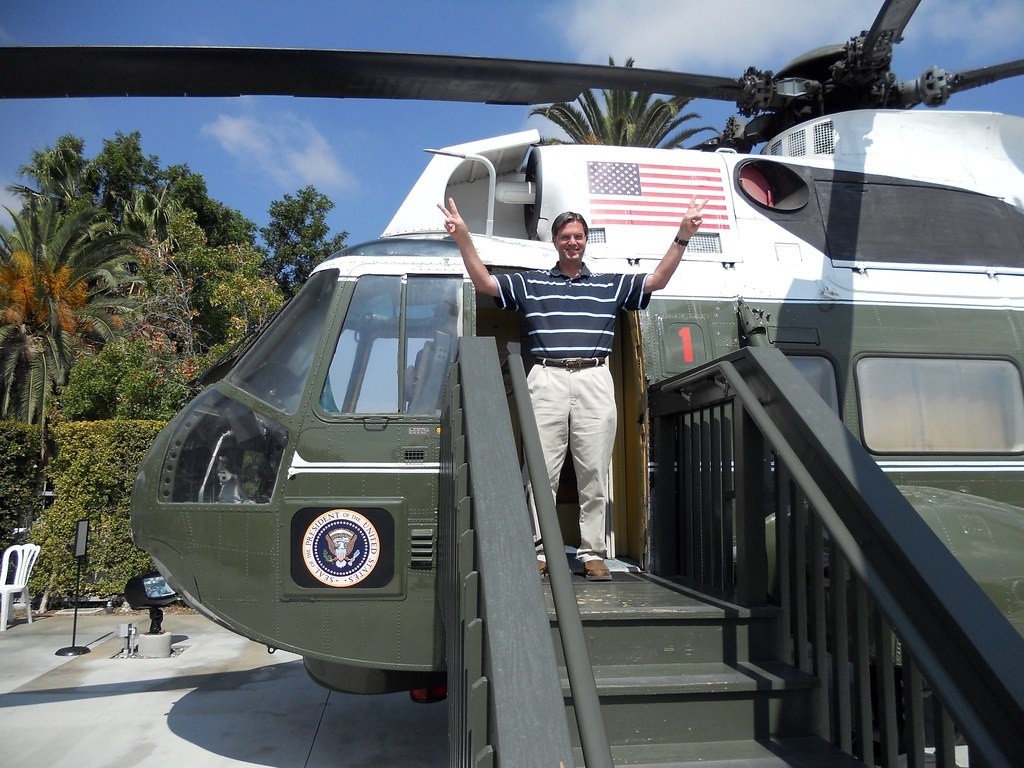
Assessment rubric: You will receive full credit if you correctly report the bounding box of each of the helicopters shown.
[0,1,1024,768]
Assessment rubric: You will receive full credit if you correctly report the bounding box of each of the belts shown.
[534,357,605,369]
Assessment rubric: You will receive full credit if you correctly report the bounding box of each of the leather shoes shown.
[538,560,545,574]
[583,559,612,580]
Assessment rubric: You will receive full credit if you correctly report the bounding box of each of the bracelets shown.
[674,236,690,247]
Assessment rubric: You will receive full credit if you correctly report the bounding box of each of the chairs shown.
[0,543,41,631]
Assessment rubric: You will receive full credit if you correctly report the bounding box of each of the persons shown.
[437,194,709,583]
